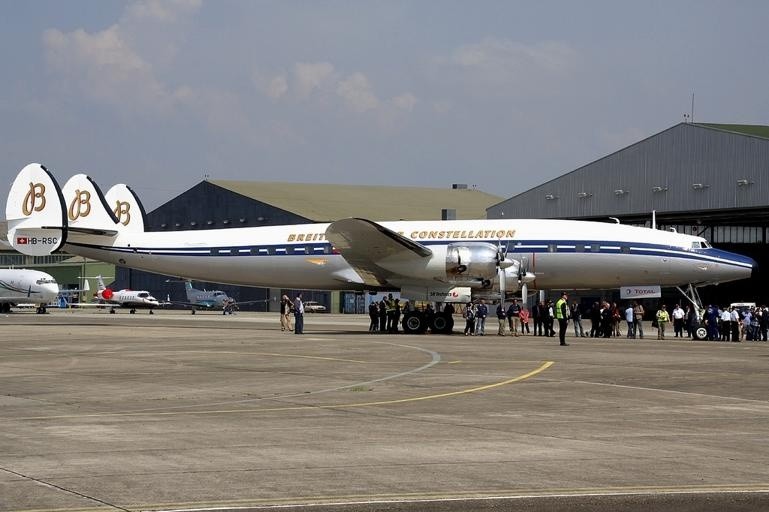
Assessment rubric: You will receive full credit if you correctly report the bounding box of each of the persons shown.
[555,293,569,346]
[444,303,455,316]
[625,302,644,338]
[700,304,769,342]
[590,301,621,338]
[425,304,432,313]
[293,291,306,335]
[497,300,530,336]
[532,300,556,337]
[403,301,409,315]
[672,304,694,337]
[464,300,488,336]
[279,294,295,332]
[656,305,670,339]
[368,293,400,331]
[571,300,586,337]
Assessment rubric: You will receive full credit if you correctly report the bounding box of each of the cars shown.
[302,300,327,313]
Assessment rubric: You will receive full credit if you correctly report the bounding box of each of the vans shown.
[725,302,758,311]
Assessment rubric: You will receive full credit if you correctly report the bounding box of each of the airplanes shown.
[162,276,275,315]
[67,269,173,315]
[5,162,760,340]
[0,268,91,315]
